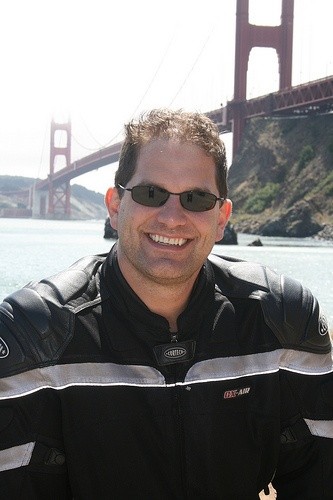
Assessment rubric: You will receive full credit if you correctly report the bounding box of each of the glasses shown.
[117,182,226,213]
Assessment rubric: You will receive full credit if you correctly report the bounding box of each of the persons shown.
[0,102,333,500]
